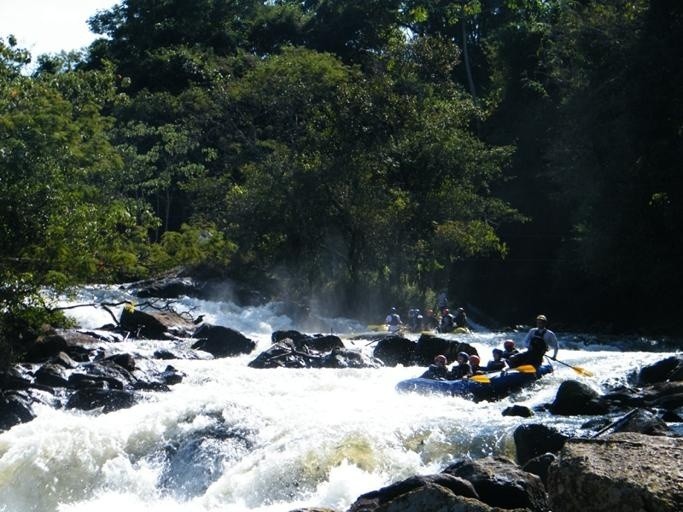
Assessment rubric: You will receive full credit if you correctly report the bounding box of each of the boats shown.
[391,356,554,402]
[366,321,438,337]
[445,326,469,334]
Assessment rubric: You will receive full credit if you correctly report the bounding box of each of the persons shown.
[485,346,510,377]
[509,314,559,369]
[501,337,519,361]
[419,354,451,382]
[467,355,487,375]
[449,352,474,379]
[382,291,469,334]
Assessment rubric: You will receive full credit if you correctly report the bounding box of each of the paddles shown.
[468,375,490,383]
[508,365,537,373]
[546,355,595,378]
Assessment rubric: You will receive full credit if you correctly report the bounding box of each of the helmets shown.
[504,340,514,349]
[536,314,546,321]
[492,348,502,355]
[426,307,463,321]
[390,307,422,315]
[469,355,480,362]
[456,351,469,360]
[434,354,447,364]
